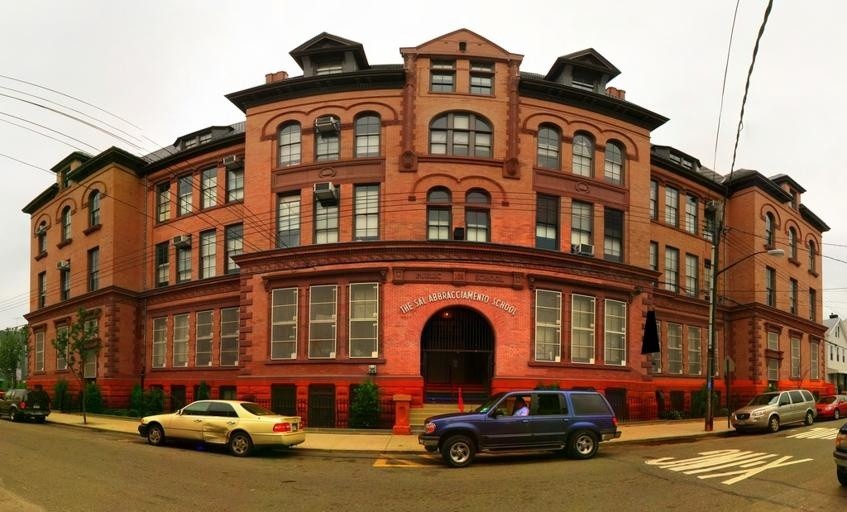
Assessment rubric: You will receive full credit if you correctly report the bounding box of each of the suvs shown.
[0,388,51,422]
[419,390,622,467]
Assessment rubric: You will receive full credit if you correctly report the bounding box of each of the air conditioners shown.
[575,243,595,256]
[56,261,68,270]
[173,236,188,245]
[36,226,44,234]
[313,181,335,200]
[316,116,337,133]
[223,155,242,167]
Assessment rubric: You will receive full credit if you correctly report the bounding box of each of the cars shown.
[832,422,847,486]
[137,400,305,456]
[816,394,847,419]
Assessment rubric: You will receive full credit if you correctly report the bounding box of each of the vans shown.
[730,389,817,430]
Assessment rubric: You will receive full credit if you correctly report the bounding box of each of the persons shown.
[512,398,530,416]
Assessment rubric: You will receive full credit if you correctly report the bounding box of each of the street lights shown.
[705,249,785,430]
